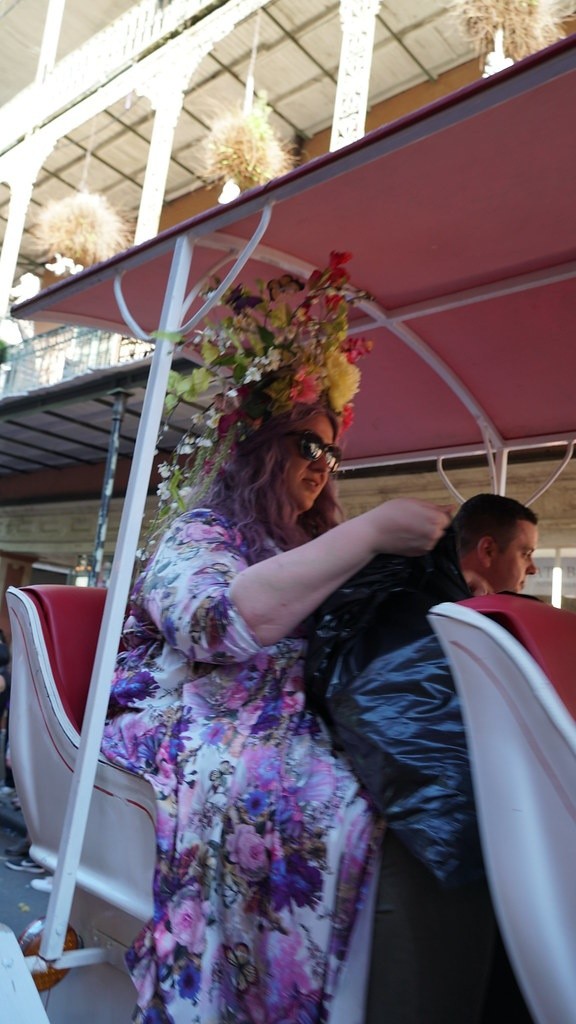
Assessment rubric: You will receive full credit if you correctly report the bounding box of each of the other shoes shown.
[3,857,46,873]
[5,839,32,857]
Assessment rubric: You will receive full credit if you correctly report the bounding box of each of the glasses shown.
[279,430,342,473]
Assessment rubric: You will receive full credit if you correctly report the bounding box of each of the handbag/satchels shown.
[303,551,478,880]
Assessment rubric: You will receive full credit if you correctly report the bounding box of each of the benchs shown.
[426,591,576,1024]
[0,584,160,1024]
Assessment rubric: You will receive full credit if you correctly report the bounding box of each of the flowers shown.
[124,247,373,599]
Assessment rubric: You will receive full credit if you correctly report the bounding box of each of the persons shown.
[454,493,539,597]
[97,360,496,1024]
[0,630,57,892]
